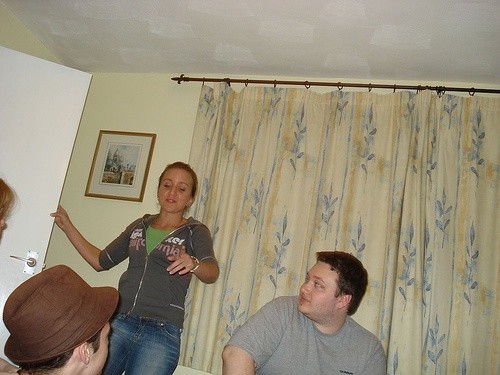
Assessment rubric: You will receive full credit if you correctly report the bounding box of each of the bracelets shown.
[190,256,200,272]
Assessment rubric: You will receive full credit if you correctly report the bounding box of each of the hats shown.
[2,264,119,365]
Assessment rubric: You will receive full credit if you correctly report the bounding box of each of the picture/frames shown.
[83,128,158,204]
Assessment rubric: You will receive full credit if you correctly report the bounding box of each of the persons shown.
[3,265,118,375]
[50,162,220,375]
[222,252,386,375]
[0,176,20,375]
